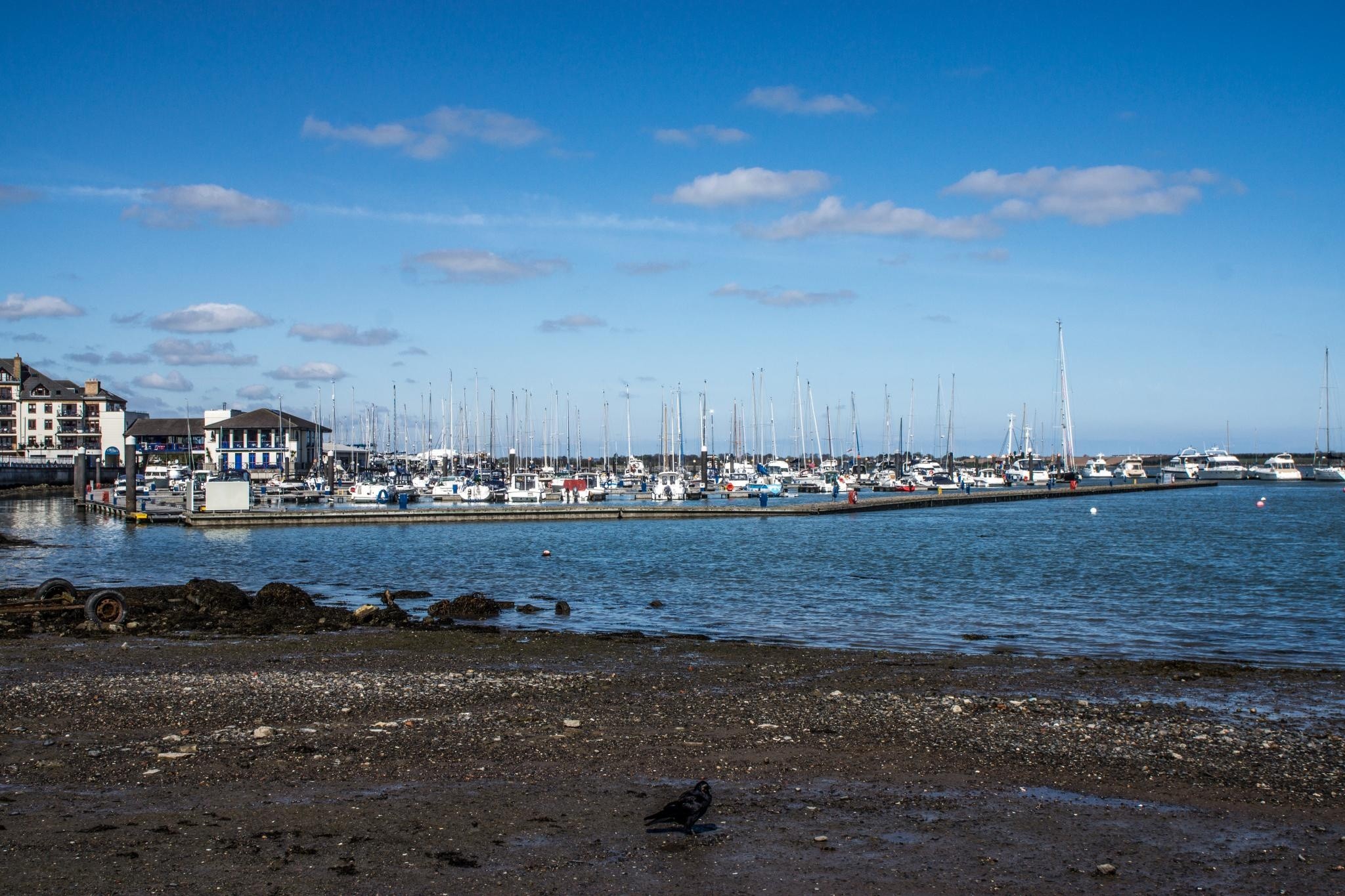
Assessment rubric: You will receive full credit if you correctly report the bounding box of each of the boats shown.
[114,474,149,496]
[1194,447,1247,479]
[1248,452,1301,481]
[1162,446,1207,479]
[144,460,254,495]
[1113,454,1147,478]
[1082,453,1114,478]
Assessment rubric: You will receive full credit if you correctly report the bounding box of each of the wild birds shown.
[644,780,712,845]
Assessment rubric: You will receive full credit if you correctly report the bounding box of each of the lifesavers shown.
[929,470,933,474]
[727,484,734,491]
[845,475,849,478]
[1073,480,1077,488]
[852,491,858,503]
[839,478,843,482]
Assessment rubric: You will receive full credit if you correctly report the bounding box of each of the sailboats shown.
[768,321,1082,492]
[539,380,783,501]
[266,368,546,506]
[1312,348,1344,481]
